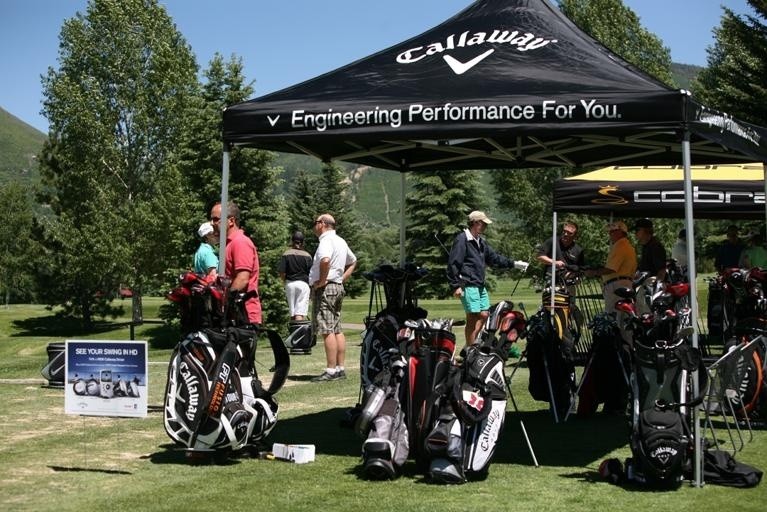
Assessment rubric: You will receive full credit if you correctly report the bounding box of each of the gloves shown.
[514,260,529,271]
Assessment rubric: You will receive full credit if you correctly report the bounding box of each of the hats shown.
[292,231,304,243]
[467,210,652,233]
[197,222,214,237]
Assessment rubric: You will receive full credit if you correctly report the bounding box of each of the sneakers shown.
[311,370,345,382]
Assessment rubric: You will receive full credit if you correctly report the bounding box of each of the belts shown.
[604,276,632,286]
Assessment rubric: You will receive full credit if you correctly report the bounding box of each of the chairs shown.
[716,329,759,452]
[697,347,734,456]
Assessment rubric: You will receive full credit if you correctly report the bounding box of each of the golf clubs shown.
[165,270,252,327]
[352,261,530,484]
[519,269,699,484]
[703,264,765,424]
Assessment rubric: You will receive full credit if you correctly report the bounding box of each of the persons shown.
[193,222,220,273]
[445,210,529,346]
[536,220,765,349]
[211,200,263,325]
[280,231,314,321]
[309,213,357,381]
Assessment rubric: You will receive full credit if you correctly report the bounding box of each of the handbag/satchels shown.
[639,409,684,490]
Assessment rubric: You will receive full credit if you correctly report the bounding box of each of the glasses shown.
[209,216,220,222]
